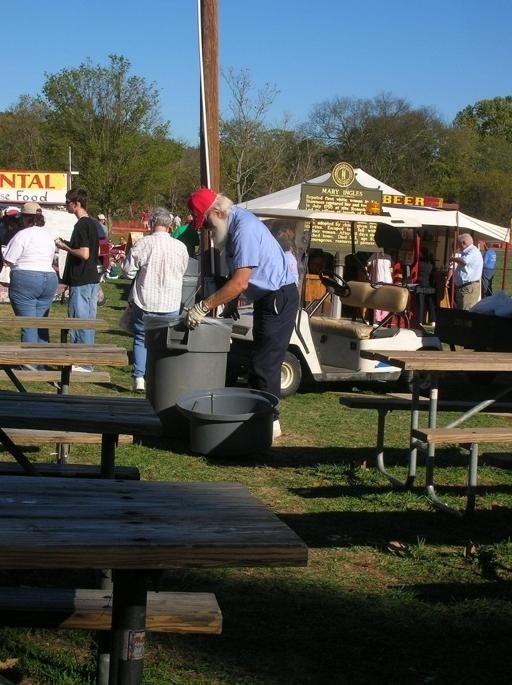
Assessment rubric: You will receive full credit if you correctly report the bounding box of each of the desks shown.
[358,349,511,521]
[0,301,166,483]
[0,474,309,683]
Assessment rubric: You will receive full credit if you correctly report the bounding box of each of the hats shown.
[97,214,107,221]
[18,202,42,215]
[187,185,217,231]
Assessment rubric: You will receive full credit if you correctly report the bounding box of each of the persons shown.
[302,230,497,338]
[183,184,305,441]
[122,187,299,389]
[0,188,127,373]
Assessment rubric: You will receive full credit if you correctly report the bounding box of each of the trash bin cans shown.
[146,316,233,447]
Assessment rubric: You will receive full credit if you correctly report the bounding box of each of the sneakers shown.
[133,378,144,393]
[72,365,93,372]
[273,420,282,437]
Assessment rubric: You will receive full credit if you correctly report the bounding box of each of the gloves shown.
[219,293,241,321]
[183,300,210,330]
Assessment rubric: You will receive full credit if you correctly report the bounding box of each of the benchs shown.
[2,580,225,684]
[338,387,511,492]
[310,279,408,340]
[409,424,511,516]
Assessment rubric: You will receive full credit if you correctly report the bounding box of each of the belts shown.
[459,281,479,289]
